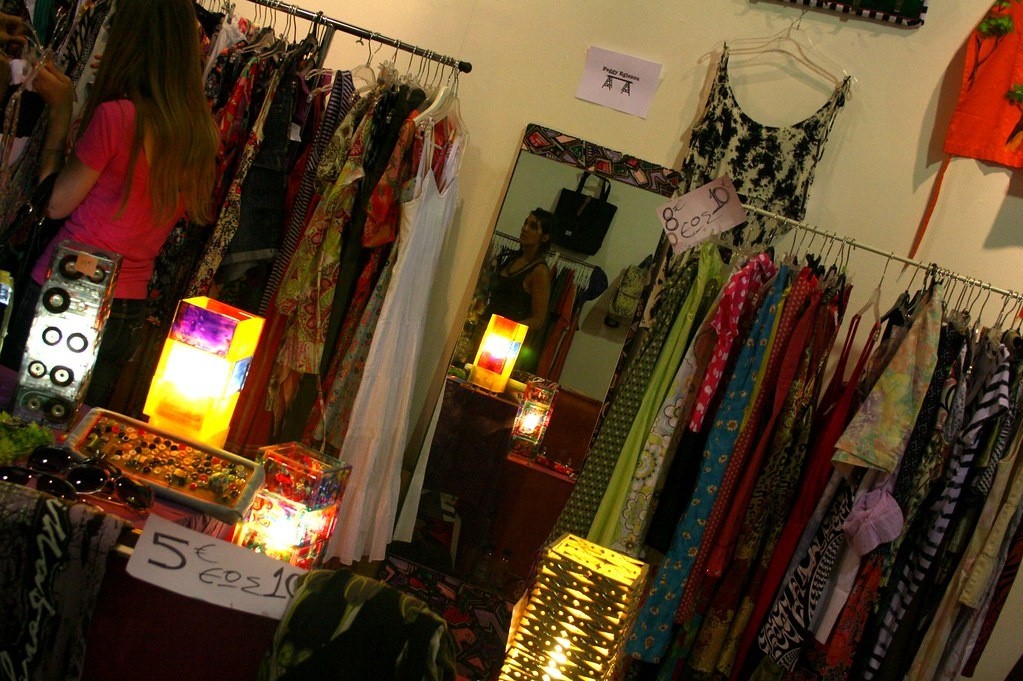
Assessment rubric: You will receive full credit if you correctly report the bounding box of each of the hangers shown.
[474,231,593,313]
[711,1,859,84]
[0,1,475,145]
[673,199,1023,378]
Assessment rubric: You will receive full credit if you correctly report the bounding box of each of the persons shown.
[0,11,32,93]
[33,0,223,411]
[475,208,555,368]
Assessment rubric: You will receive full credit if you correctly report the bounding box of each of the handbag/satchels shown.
[552,171,618,256]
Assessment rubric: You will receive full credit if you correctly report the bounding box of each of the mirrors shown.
[391,123,683,595]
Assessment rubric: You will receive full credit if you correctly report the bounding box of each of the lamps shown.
[505,376,561,462]
[467,313,529,394]
[495,532,650,681]
[229,440,353,574]
[144,297,267,452]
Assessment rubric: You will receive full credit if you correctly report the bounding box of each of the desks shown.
[0,364,234,542]
[486,449,580,575]
[436,373,519,580]
